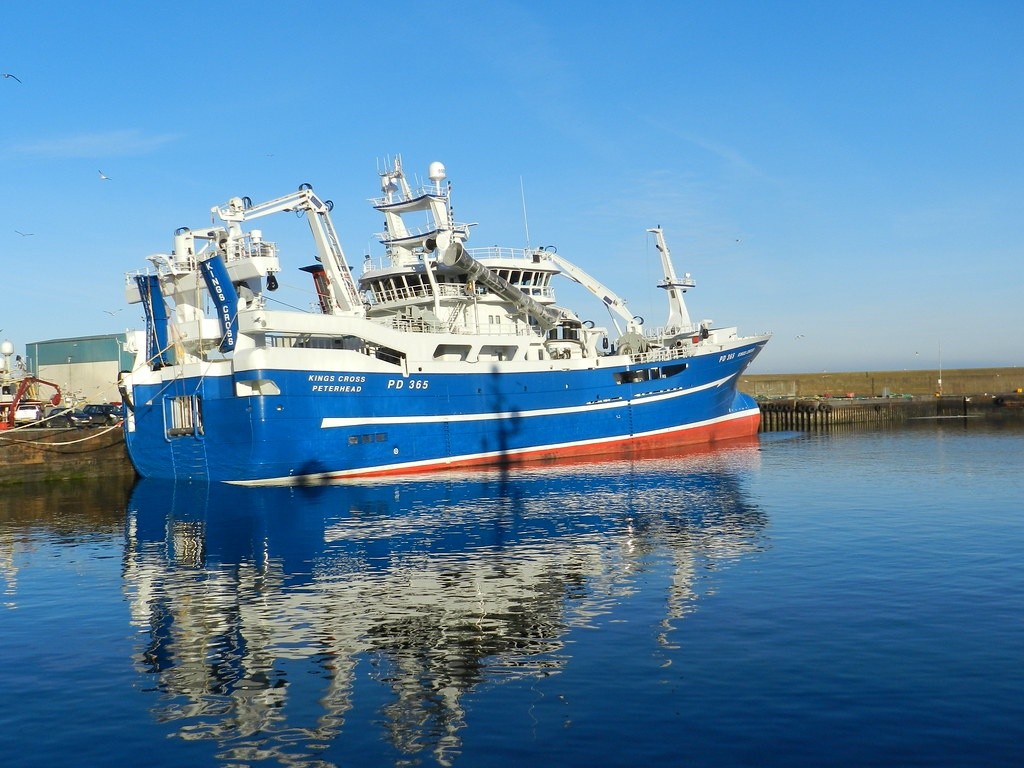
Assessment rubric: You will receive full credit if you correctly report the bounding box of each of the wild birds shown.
[14,230,35,237]
[735,239,744,243]
[102,309,124,317]
[97,169,112,182]
[0,72,22,85]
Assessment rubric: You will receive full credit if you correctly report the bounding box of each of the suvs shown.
[14,402,43,427]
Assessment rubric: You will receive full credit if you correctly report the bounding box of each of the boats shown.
[117,152,774,488]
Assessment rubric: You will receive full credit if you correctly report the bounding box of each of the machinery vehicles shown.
[0,376,62,430]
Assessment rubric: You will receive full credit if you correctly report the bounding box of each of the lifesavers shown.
[757,401,833,413]
[875,404,880,412]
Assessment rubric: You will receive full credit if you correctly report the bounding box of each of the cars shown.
[46,407,93,428]
[83,404,123,426]
